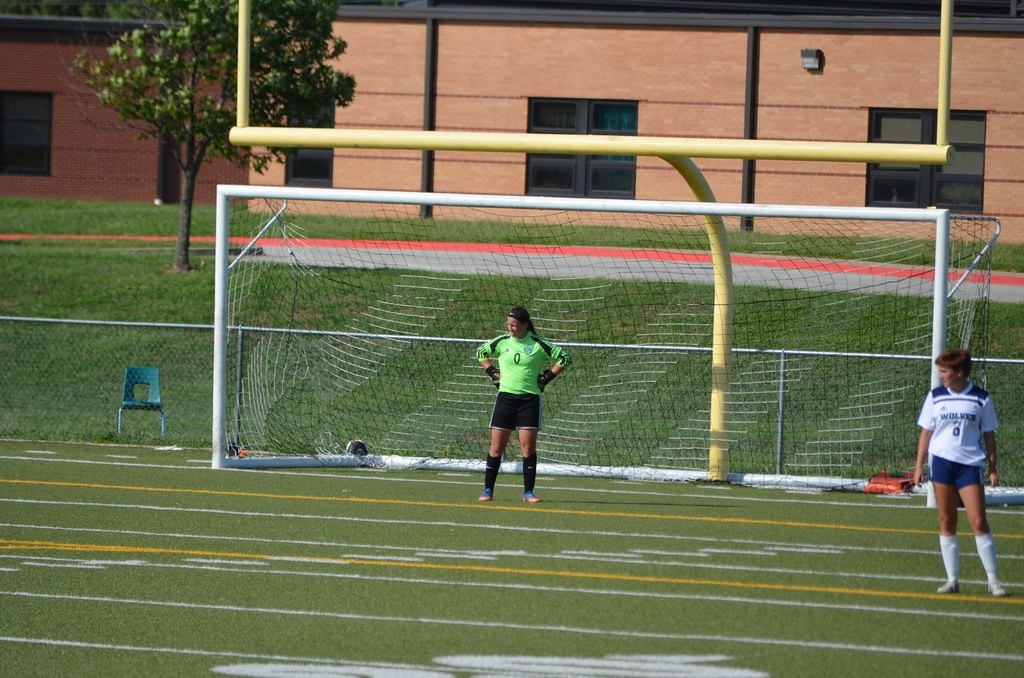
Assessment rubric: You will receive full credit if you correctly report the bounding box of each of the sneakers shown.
[523,491,541,503]
[988,583,1007,597]
[478,489,493,500]
[937,583,959,594]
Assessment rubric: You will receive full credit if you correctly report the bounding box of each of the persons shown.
[476,307,573,503]
[913,349,1006,597]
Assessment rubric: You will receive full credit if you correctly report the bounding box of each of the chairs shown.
[117,366,166,437]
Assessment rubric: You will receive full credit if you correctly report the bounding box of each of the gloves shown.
[538,370,556,392]
[485,365,500,389]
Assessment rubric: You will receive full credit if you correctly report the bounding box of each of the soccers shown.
[346,438,368,457]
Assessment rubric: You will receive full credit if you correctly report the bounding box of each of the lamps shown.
[801,49,823,70]
